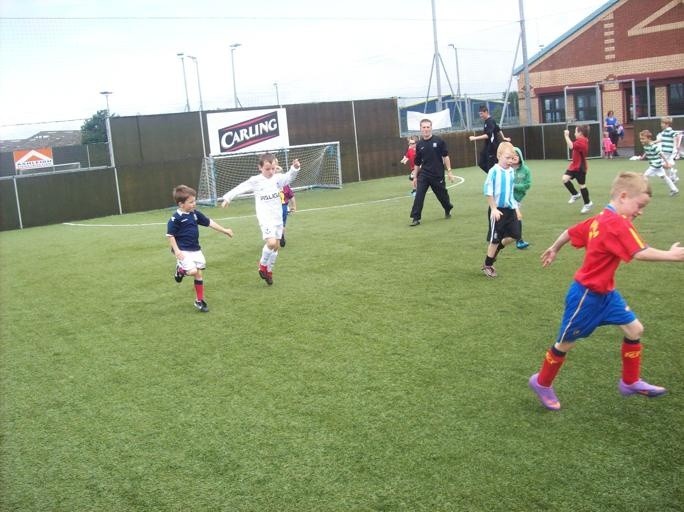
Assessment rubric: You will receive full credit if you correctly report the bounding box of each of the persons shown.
[275,158,296,247]
[564,109,684,215]
[399,106,533,279]
[528,169,684,410]
[218,153,301,286]
[166,183,234,312]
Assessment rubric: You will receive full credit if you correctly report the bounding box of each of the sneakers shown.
[580,200,595,214]
[528,371,561,411]
[280,236,285,247]
[257,261,273,285]
[410,189,417,196]
[568,192,582,204]
[603,151,621,159]
[516,240,529,248]
[667,188,680,197]
[193,298,209,312]
[480,260,498,279]
[174,262,184,282]
[619,377,668,397]
[444,208,451,219]
[409,218,421,226]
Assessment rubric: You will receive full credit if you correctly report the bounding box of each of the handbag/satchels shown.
[609,125,619,144]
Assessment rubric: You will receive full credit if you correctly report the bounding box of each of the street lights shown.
[448,43,465,130]
[187,56,204,111]
[177,52,191,113]
[229,43,244,109]
[272,82,280,105]
[99,89,113,115]
